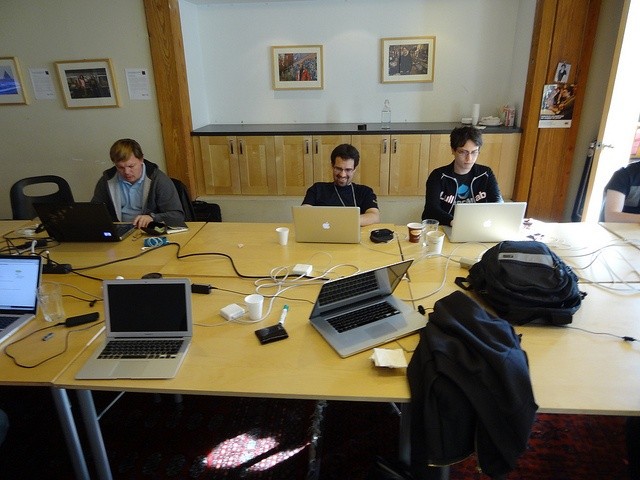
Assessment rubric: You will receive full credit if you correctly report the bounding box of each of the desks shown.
[599,221,640,246]
[0,218,205,480]
[53,221,640,480]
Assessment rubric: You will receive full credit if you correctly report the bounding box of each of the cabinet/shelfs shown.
[431,133,523,202]
[349,134,430,200]
[192,135,278,197]
[275,134,352,198]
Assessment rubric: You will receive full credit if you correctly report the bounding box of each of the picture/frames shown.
[0,56,30,105]
[270,45,325,90]
[553,61,572,83]
[380,35,436,83]
[54,58,121,111]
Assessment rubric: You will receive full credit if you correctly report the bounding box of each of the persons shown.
[554,86,573,119]
[75,72,87,94]
[397,46,413,74]
[420,123,505,227]
[294,63,313,82]
[557,64,568,82]
[299,143,380,227]
[87,138,186,231]
[598,159,640,224]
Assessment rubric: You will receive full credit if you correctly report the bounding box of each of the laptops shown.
[0,255,41,343]
[308,259,425,359]
[74,277,194,382]
[291,205,362,245]
[31,201,137,241]
[441,201,530,244]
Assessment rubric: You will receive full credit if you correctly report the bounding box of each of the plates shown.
[480,122,502,127]
[473,125,487,129]
[461,118,472,125]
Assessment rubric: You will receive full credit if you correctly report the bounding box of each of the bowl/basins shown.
[481,116,500,124]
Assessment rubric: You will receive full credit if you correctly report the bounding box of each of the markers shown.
[278,305,288,325]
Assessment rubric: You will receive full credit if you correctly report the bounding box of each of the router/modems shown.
[293,263,312,276]
[220,303,245,321]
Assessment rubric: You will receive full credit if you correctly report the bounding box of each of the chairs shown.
[166,176,195,220]
[10,174,74,220]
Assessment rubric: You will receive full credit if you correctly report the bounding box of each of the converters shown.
[64,311,99,326]
[192,282,212,294]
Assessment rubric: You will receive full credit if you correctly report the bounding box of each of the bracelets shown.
[148,212,156,221]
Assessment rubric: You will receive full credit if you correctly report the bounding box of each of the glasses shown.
[456,148,480,156]
[334,166,355,174]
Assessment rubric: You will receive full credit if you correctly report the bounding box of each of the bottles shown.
[381,98,392,129]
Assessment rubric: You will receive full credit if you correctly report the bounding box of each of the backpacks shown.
[455,240,587,327]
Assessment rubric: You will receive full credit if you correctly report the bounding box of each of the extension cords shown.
[43,263,71,275]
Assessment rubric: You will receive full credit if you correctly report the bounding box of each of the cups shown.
[426,231,445,255]
[407,222,424,243]
[421,219,440,246]
[472,104,480,126]
[275,227,290,245]
[35,283,65,322]
[244,294,264,320]
